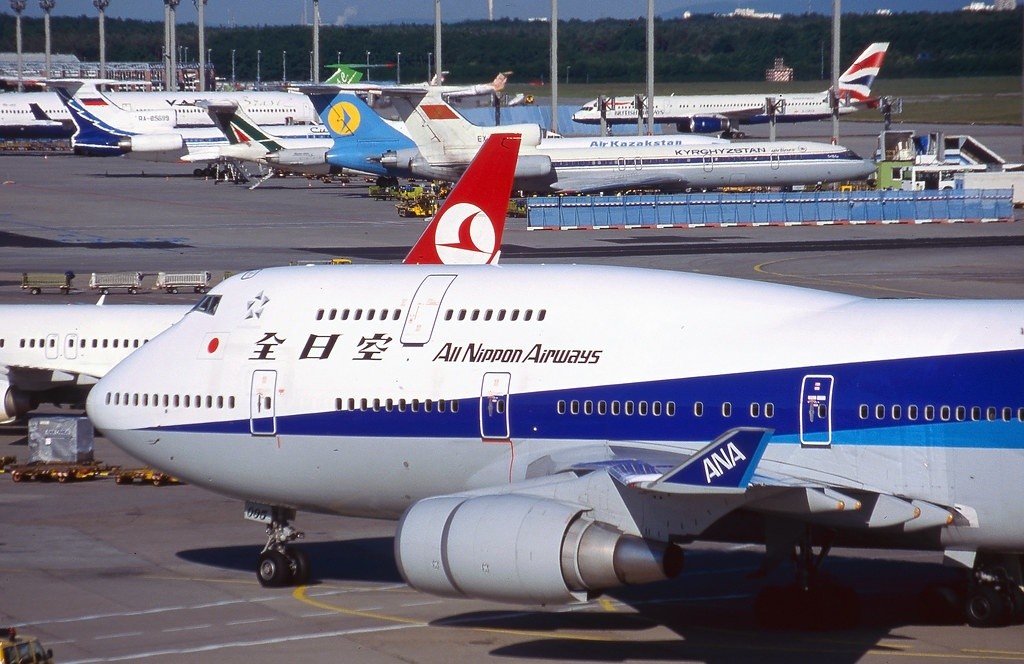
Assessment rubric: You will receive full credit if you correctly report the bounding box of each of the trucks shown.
[396,194,439,217]
[505,199,530,217]
[368,184,407,201]
[398,186,424,200]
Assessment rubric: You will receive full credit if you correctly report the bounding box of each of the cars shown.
[530,79,545,86]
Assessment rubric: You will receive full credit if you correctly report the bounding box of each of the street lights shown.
[162,45,166,64]
[257,50,261,86]
[282,51,287,82]
[310,50,314,82]
[186,47,188,63]
[338,52,341,70]
[180,46,183,64]
[397,52,401,85]
[208,49,212,67]
[367,51,371,82]
[232,49,235,83]
[428,52,432,84]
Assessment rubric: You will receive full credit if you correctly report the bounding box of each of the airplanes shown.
[0,295,196,425]
[86,134,1023,628]
[0,70,882,194]
